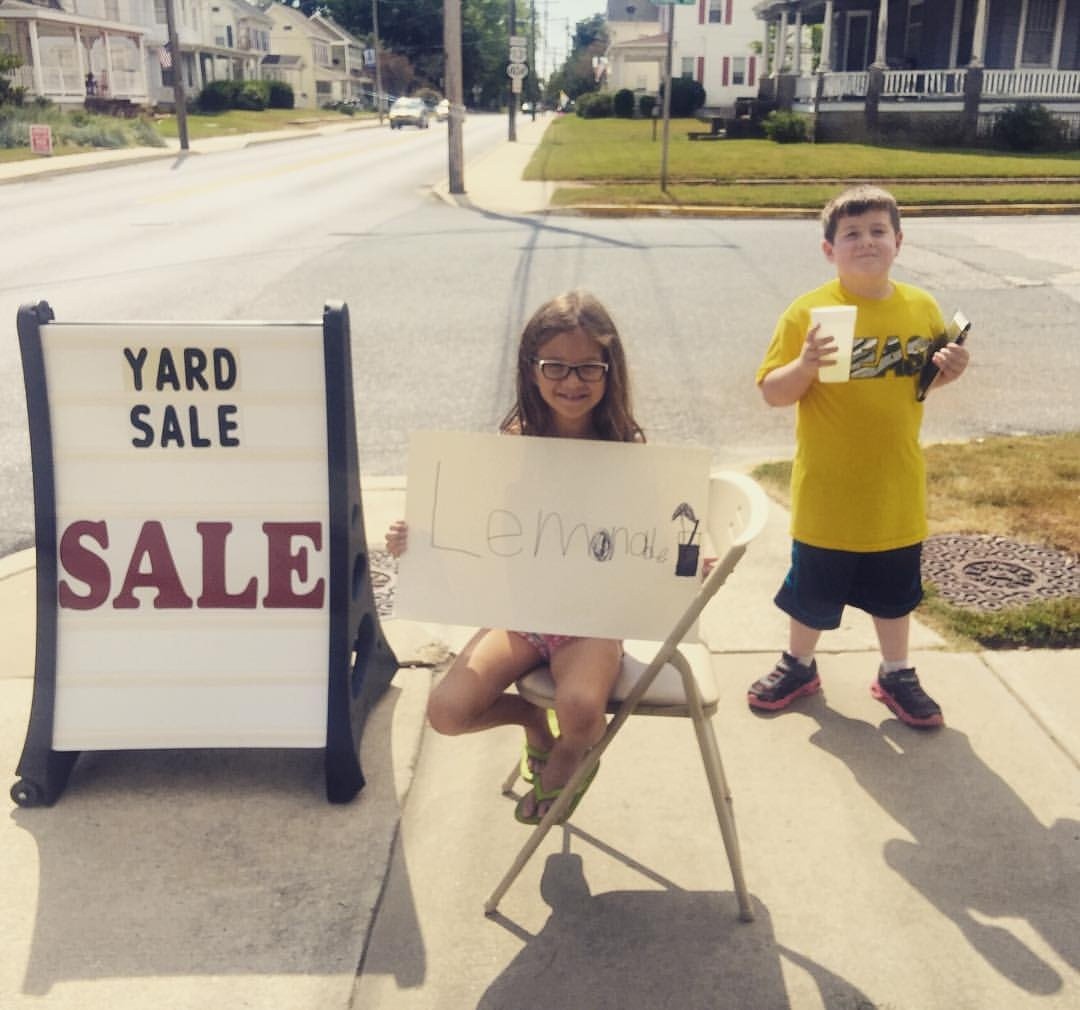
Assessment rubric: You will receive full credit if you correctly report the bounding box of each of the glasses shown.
[531,359,610,382]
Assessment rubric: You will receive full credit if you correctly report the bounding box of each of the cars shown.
[522,104,532,113]
[389,96,428,129]
[436,99,466,122]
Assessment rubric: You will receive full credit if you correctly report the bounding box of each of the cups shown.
[812,305,857,384]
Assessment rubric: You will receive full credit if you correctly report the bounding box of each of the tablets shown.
[916,315,970,401]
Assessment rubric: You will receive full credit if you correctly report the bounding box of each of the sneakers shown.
[878,664,944,727]
[748,650,819,709]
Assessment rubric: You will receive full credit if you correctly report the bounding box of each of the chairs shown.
[483,472,770,924]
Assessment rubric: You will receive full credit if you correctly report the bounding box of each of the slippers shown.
[521,710,560,782]
[515,748,598,825]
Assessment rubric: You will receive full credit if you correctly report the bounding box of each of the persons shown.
[747,190,971,725]
[383,291,734,824]
